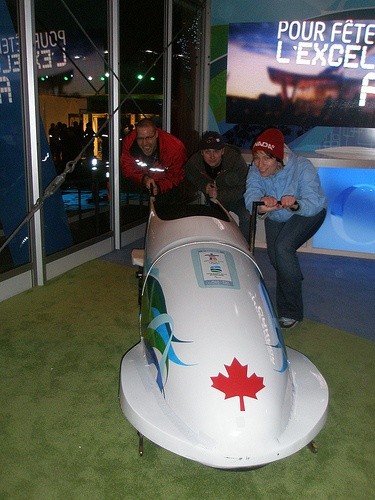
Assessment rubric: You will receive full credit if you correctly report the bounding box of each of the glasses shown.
[136,133,155,142]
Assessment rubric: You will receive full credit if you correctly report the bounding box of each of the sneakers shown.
[276,316,299,330]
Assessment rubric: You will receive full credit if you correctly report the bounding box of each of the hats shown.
[200,131,226,151]
[253,129,285,161]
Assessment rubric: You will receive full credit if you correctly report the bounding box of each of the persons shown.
[245,128,327,330]
[48,112,139,166]
[185,131,250,242]
[120,117,187,197]
